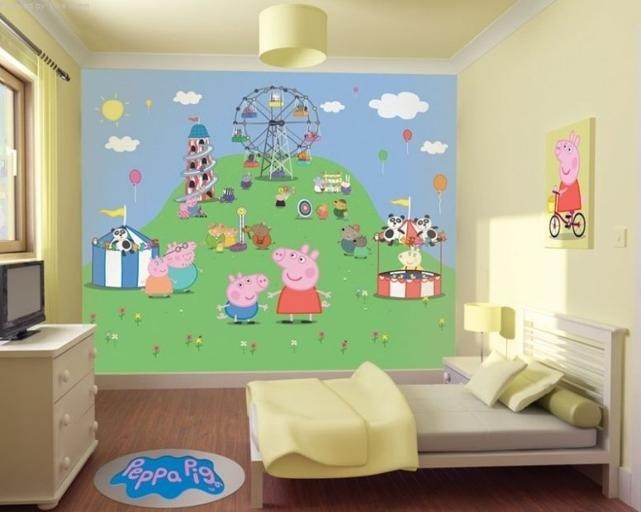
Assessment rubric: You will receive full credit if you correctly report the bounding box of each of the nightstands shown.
[443,356,489,383]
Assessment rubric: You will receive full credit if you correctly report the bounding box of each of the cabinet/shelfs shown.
[0,323,99,511]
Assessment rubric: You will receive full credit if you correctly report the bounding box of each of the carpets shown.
[93,448,246,508]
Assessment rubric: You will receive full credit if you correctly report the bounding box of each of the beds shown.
[245,308,631,508]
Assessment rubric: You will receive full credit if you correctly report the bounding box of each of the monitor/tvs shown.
[0,258,46,340]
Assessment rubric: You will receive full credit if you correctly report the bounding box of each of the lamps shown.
[464,302,501,368]
[258,3,328,68]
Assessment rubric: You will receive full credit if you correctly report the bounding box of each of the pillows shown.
[498,355,563,413]
[537,386,602,428]
[463,350,528,408]
